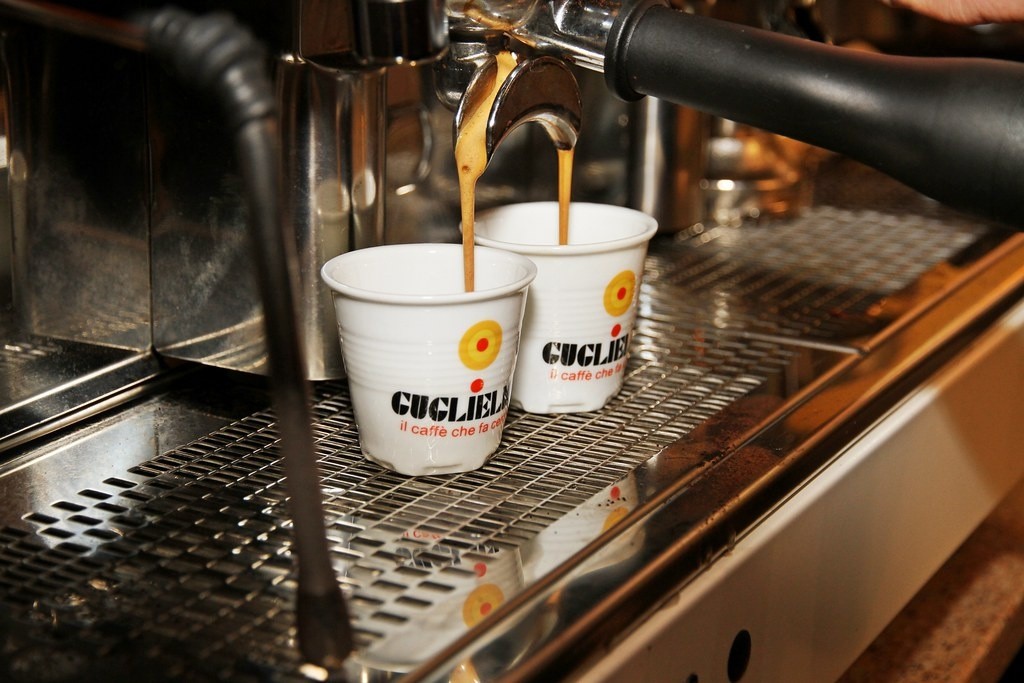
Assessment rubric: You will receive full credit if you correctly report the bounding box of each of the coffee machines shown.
[1,0,663,383]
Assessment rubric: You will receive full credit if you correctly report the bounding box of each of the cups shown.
[319,242,539,477]
[459,200,659,414]
